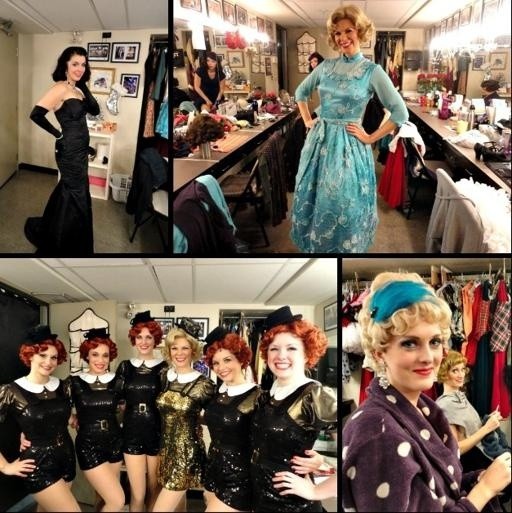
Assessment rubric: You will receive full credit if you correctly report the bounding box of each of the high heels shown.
[474,141,507,162]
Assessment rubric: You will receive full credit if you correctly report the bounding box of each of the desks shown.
[404,97,512,195]
[173,107,300,205]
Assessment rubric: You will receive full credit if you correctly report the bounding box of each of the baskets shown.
[109,174,132,203]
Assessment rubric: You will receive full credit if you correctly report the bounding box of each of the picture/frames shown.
[88,42,140,97]
[323,300,337,331]
[181,0,275,39]
[153,317,211,379]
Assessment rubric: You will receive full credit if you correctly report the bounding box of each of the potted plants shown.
[185,117,223,159]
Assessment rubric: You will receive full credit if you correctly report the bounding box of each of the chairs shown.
[374,109,488,253]
[220,113,317,246]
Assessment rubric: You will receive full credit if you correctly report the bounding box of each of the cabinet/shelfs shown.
[58,133,114,200]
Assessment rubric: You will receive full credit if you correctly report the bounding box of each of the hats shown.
[84,328,110,339]
[204,326,231,355]
[21,325,57,345]
[260,306,302,340]
[174,318,200,339]
[130,311,155,327]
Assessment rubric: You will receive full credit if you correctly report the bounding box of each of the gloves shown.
[30,105,65,162]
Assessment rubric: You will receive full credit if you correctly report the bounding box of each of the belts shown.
[128,403,156,414]
[81,419,119,432]
[33,434,69,447]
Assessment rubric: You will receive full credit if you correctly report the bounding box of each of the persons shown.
[290,5,410,254]
[24,46,101,253]
[436,350,512,513]
[192,54,227,112]
[307,52,326,73]
[341,271,512,513]
[0,319,337,513]
